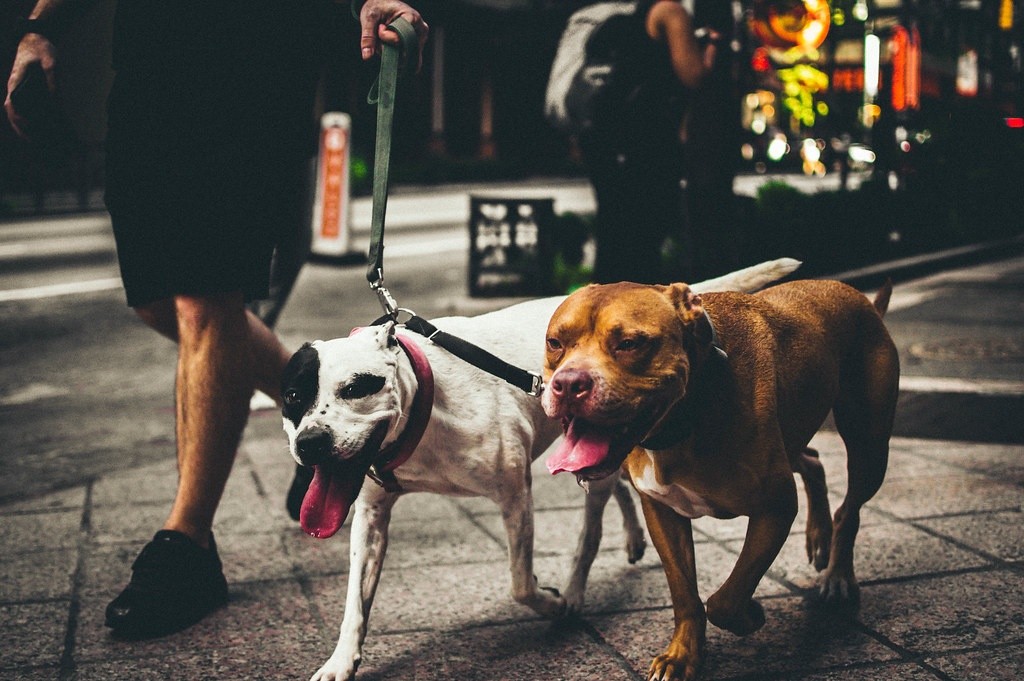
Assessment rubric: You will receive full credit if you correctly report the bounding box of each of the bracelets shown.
[18,18,54,39]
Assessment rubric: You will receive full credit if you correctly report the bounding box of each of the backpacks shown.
[543,0,642,132]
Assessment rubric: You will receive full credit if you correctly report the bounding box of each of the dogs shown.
[282,257,900,681]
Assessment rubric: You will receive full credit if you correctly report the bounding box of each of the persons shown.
[3,2,431,639]
[578,0,720,284]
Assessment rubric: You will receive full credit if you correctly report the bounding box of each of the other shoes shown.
[103,529,229,631]
[286,464,315,521]
